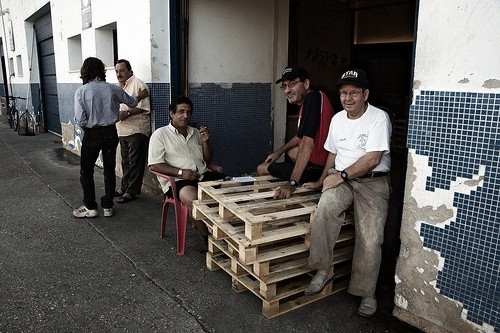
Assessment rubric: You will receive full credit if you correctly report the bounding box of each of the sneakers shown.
[103,207,115,217]
[72,205,98,218]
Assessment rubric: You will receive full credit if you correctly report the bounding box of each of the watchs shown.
[126,110,131,116]
[288,179,297,187]
[178,168,183,179]
[340,170,348,182]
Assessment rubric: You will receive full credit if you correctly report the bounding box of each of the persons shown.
[257,64,335,201]
[72,57,149,218]
[146,96,233,249]
[109,59,151,204]
[301,67,392,318]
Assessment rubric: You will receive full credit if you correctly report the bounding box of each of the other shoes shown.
[304,267,335,296]
[114,191,124,197]
[116,194,136,203]
[357,297,377,317]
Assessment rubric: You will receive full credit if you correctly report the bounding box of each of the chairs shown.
[149,164,224,256]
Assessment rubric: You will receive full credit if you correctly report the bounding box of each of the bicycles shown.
[1,95,27,132]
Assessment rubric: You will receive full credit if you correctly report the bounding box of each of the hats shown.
[337,68,370,88]
[276,65,301,84]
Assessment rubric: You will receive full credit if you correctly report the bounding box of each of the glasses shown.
[280,80,300,90]
[338,90,364,97]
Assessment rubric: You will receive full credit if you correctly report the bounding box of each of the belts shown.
[357,171,388,179]
